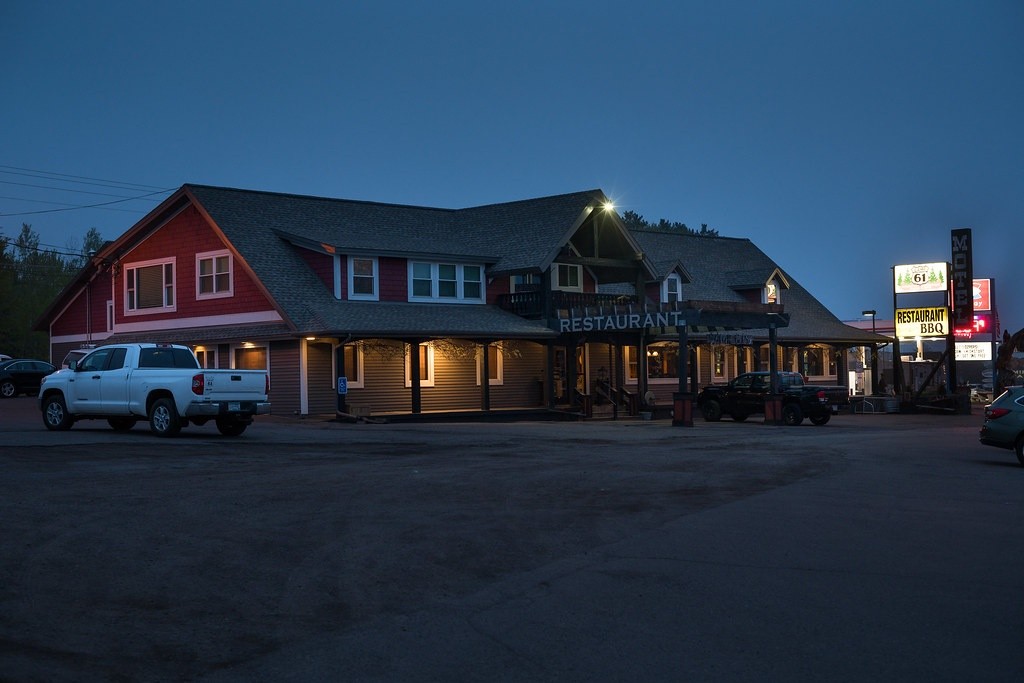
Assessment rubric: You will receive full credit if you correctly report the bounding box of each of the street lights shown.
[861,310,876,332]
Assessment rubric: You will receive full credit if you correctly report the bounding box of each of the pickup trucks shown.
[38,342,272,438]
[696,371,851,426]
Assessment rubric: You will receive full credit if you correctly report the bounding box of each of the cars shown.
[978,384,1024,469]
[0,354,58,400]
